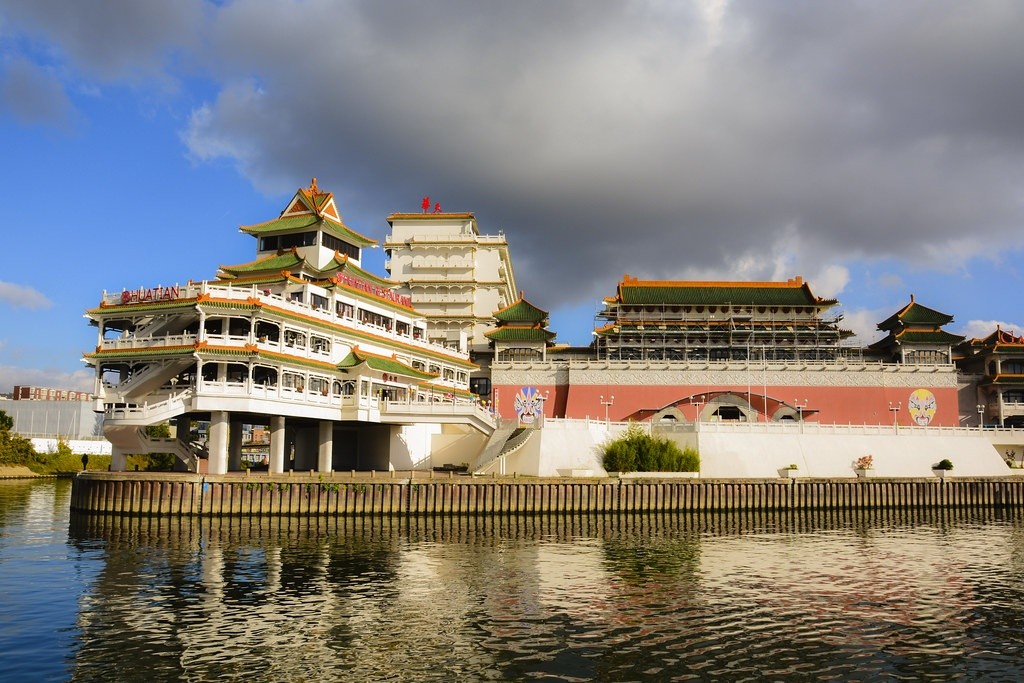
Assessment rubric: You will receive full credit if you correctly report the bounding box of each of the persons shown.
[82,452,89,470]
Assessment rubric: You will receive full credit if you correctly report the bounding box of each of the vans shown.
[971,424,1003,431]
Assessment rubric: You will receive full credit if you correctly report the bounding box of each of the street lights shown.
[889,401,902,426]
[794,398,808,420]
[976,404,985,428]
[474,384,479,406]
[689,395,705,421]
[535,389,549,413]
[599,395,615,420]
